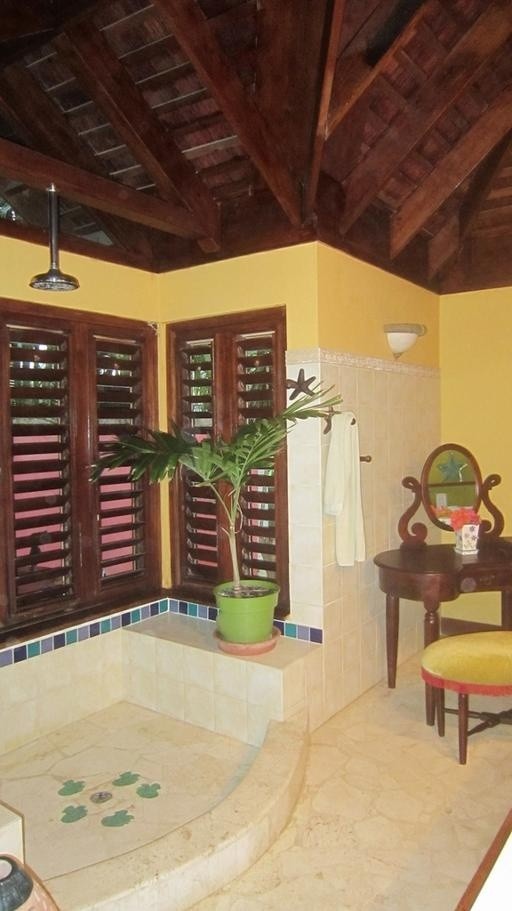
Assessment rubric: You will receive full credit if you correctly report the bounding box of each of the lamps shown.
[385,321,428,360]
[30,186,81,293]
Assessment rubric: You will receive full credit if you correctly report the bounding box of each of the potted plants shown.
[83,376,345,656]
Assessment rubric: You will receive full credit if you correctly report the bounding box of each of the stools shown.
[415,627,512,761]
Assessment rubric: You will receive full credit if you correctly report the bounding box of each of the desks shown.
[373,539,512,727]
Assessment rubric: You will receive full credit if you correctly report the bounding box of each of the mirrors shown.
[399,440,503,551]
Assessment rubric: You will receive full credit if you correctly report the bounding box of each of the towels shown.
[324,410,366,567]
